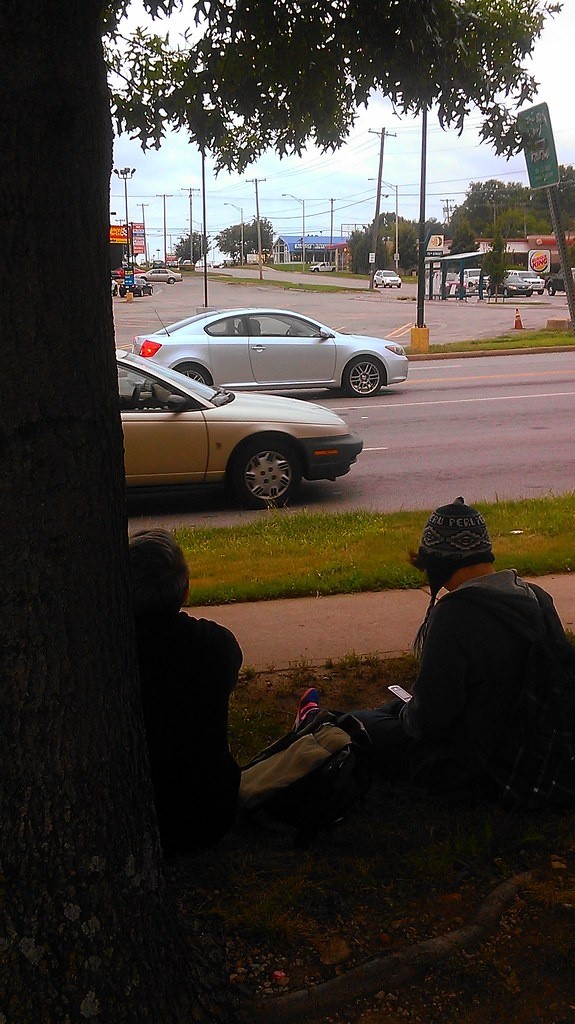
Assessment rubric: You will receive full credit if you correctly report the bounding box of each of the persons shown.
[129,530,242,859]
[250,318,261,336]
[292,497,575,813]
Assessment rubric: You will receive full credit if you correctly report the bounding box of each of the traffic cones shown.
[510,308,526,331]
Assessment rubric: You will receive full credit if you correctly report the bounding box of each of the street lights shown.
[113,167,137,268]
[282,194,306,263]
[368,178,399,273]
[223,202,244,266]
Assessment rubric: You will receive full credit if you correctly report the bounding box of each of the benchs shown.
[425,293,505,303]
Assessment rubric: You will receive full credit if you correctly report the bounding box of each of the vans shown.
[152,260,165,269]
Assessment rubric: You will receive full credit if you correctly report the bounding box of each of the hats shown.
[411,495,495,662]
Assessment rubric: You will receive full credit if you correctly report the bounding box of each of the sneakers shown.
[294,688,318,729]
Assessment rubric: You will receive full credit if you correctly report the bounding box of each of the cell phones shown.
[387,685,412,702]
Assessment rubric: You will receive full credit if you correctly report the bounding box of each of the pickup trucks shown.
[309,262,336,272]
[503,269,546,295]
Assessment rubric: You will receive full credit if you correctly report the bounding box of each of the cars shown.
[487,274,534,298]
[139,268,183,285]
[166,260,179,268]
[456,269,492,293]
[122,266,145,274]
[115,347,363,510]
[374,269,402,288]
[435,270,467,294]
[183,260,194,265]
[110,275,118,296]
[544,267,575,296]
[119,277,154,298]
[213,262,220,268]
[111,270,121,280]
[124,308,410,398]
